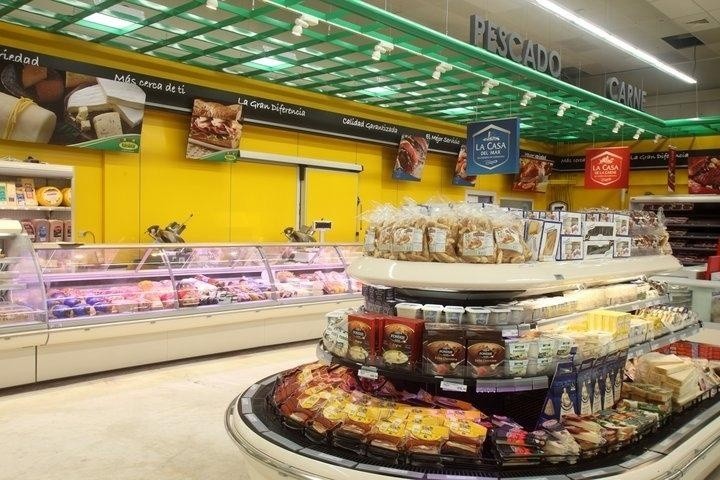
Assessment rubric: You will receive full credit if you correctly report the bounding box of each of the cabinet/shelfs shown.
[223,251,718,478]
[629,195,718,263]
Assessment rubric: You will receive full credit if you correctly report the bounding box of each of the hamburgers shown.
[404,133,429,157]
[396,140,420,175]
[188,99,243,154]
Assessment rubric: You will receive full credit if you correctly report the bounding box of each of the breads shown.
[360,200,533,265]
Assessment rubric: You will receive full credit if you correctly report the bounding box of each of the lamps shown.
[481,78,502,97]
[586,110,662,146]
[372,38,394,63]
[291,12,322,38]
[432,60,454,80]
[519,91,570,118]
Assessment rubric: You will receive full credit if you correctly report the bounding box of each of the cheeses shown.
[65,74,147,142]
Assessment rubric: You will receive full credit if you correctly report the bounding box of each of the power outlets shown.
[0,159,75,251]
